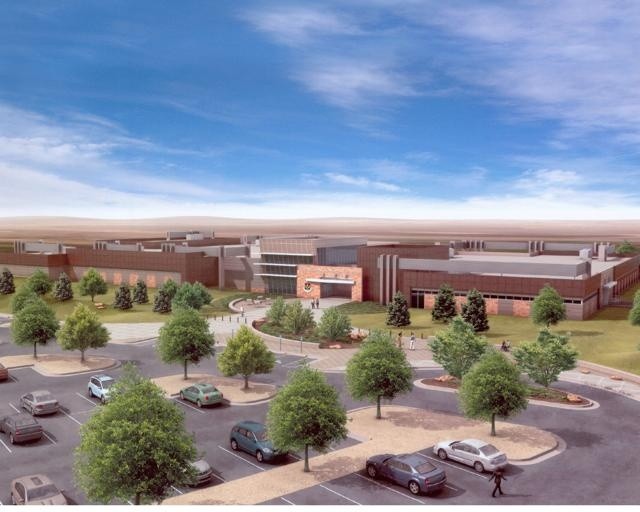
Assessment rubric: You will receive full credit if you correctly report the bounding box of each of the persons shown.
[310,297,315,308]
[409,332,416,351]
[501,341,505,350]
[488,466,508,498]
[398,330,403,347]
[316,298,320,309]
[504,338,511,351]
